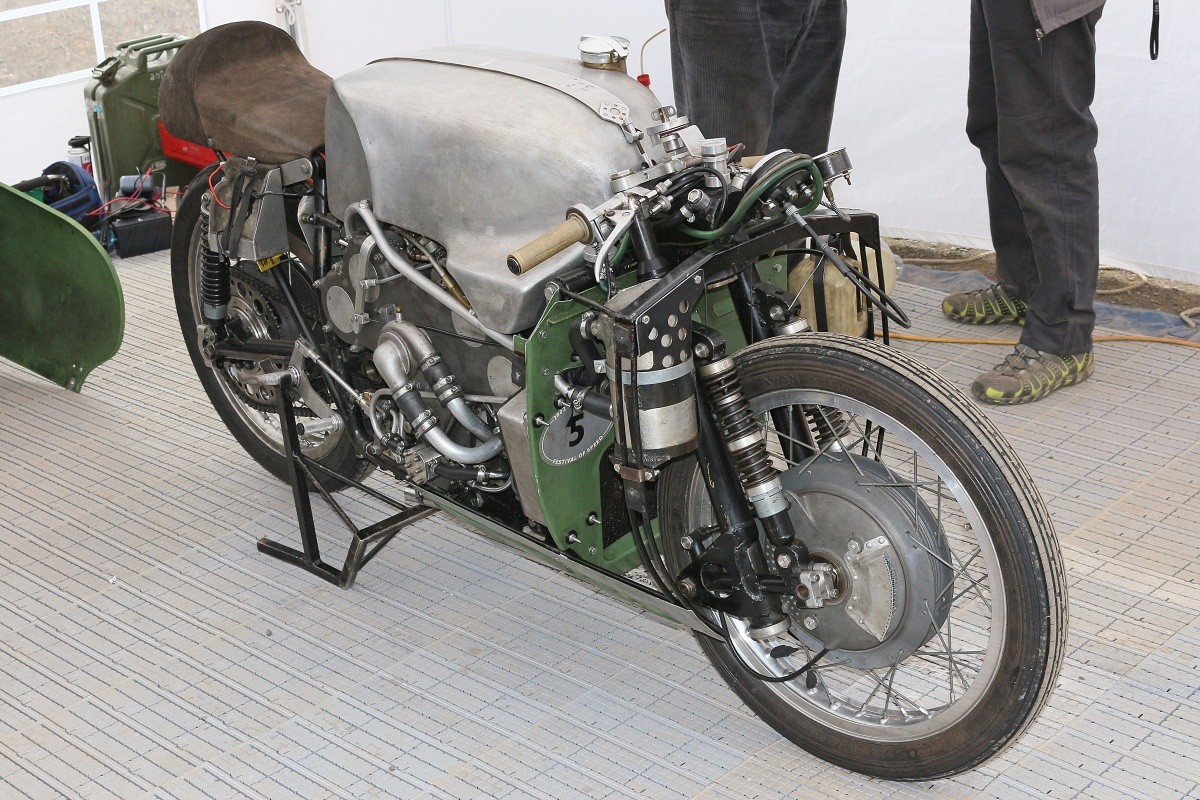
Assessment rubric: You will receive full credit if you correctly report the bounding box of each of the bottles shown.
[67,134,93,178]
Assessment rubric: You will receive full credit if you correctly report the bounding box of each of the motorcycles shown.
[158,19,1068,781]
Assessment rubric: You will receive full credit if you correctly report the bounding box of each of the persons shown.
[940,0,1106,405]
[666,0,848,160]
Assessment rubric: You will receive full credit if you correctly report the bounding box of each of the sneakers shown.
[941,282,1028,327]
[971,339,1095,404]
